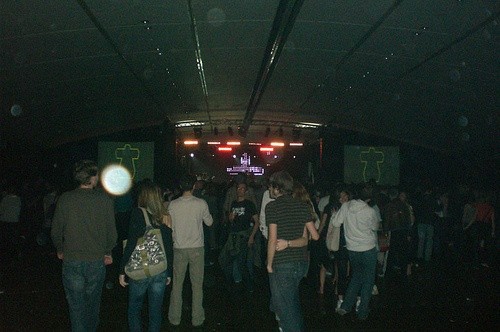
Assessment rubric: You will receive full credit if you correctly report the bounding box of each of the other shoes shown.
[336,299,344,312]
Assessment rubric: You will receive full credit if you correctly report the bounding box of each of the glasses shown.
[236,186,246,191]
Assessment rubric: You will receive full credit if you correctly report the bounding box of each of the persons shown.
[331,183,381,321]
[161,173,213,328]
[265,170,320,332]
[275,177,321,321]
[118,181,172,332]
[49,159,118,332]
[0,167,500,310]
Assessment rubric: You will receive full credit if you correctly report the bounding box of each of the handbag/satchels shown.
[123,206,167,280]
